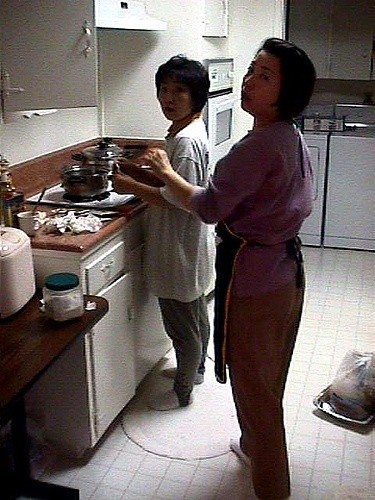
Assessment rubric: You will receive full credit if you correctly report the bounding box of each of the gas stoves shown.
[26,165,155,208]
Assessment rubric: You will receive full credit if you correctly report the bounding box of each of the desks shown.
[1,288,109,500]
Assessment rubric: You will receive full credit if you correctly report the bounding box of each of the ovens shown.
[98,27,234,168]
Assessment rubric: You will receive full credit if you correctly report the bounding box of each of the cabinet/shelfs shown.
[25,134,374,462]
[288,1,375,81]
[1,0,228,124]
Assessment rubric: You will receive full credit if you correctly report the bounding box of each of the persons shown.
[111,56,213,410]
[135,37,317,500]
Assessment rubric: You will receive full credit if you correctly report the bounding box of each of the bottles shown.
[42,272,84,321]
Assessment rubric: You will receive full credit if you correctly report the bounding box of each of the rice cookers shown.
[0,227,36,319]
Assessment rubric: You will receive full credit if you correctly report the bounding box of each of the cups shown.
[17,211,42,236]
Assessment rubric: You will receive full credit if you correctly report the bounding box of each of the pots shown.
[70,137,149,178]
[61,167,111,198]
